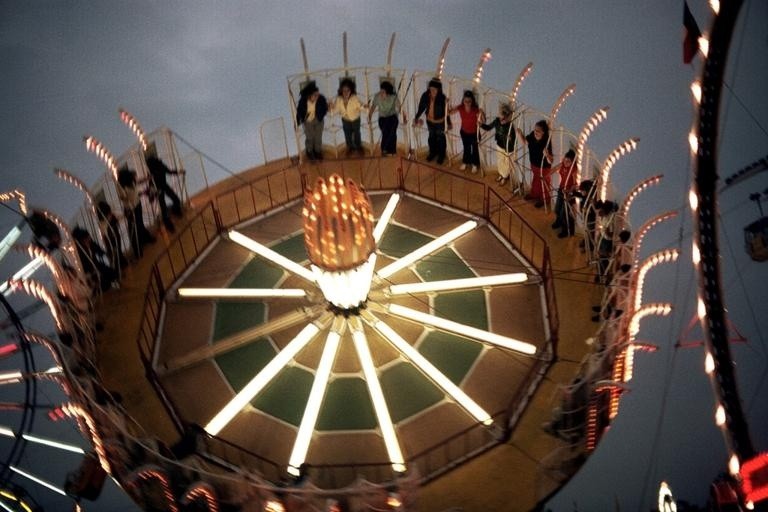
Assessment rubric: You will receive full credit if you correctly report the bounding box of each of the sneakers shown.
[426,153,447,165]
[460,163,478,173]
[308,154,325,161]
[551,220,561,230]
[592,305,601,313]
[557,230,568,239]
[523,194,533,202]
[534,201,543,210]
[348,147,365,159]
[382,150,397,158]
[592,315,600,322]
[135,207,184,260]
[495,175,507,187]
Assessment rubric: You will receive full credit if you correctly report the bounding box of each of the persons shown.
[169,442,412,512]
[543,199,632,445]
[294,76,597,254]
[60,155,185,476]
